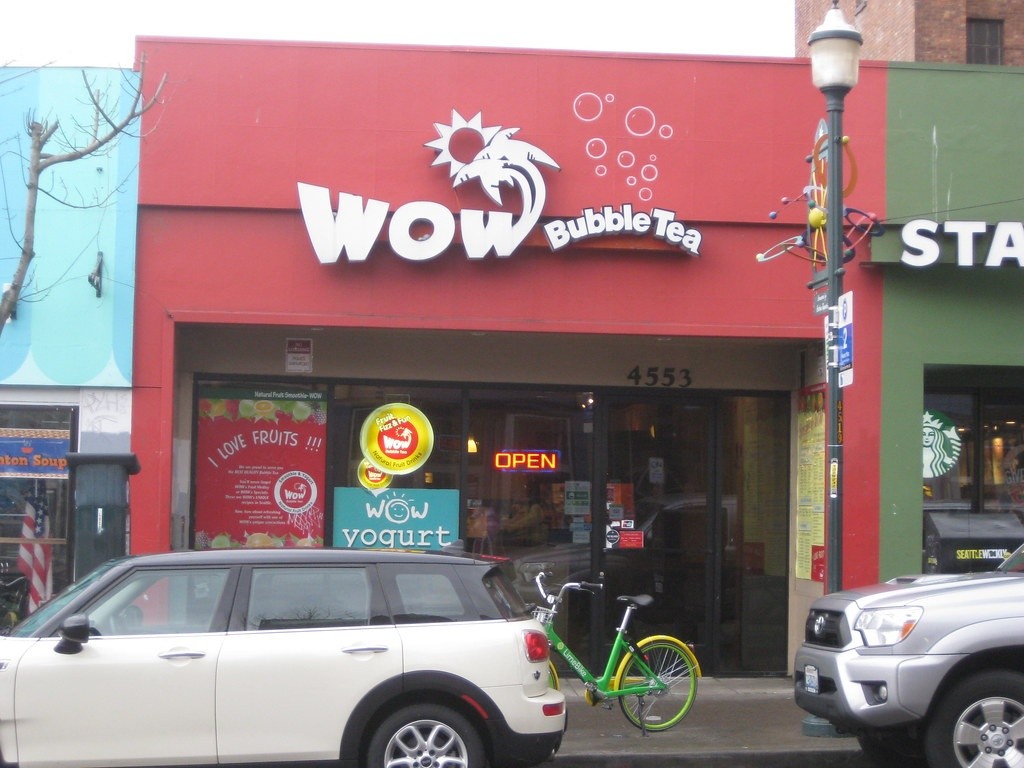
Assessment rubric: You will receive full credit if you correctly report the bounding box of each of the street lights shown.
[806,6,865,594]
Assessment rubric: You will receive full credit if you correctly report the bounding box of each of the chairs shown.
[191,591,425,625]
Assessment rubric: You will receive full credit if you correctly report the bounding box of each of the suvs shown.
[791,536,1023,766]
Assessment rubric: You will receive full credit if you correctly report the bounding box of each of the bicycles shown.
[534,568,704,740]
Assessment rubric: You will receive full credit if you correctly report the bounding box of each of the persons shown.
[497,481,549,548]
[923,411,961,478]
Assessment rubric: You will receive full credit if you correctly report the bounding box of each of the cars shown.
[0,540,570,767]
